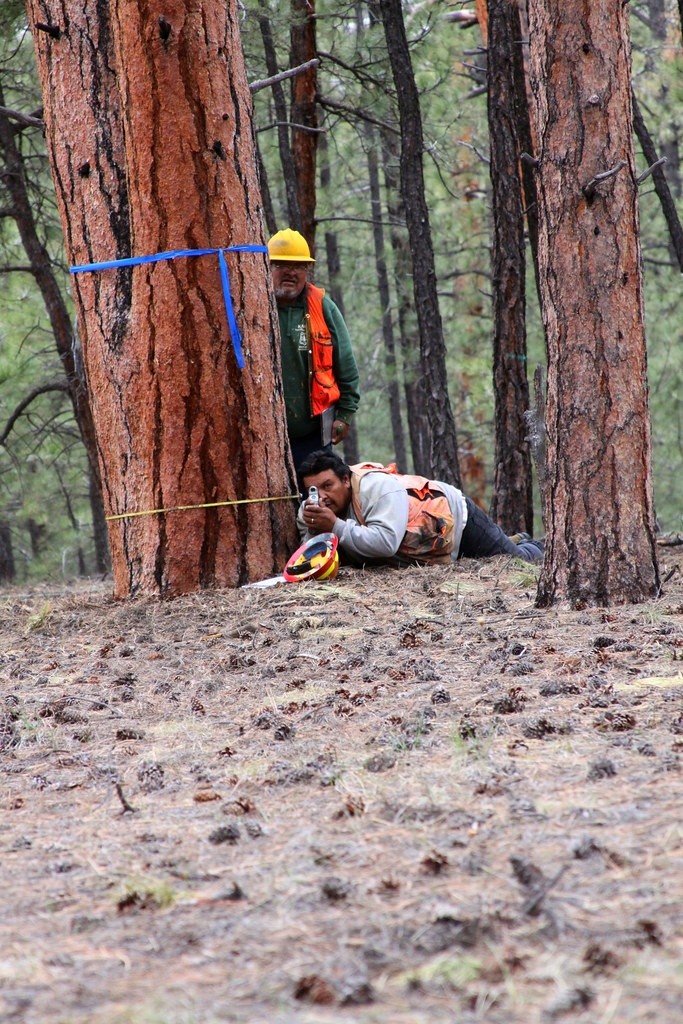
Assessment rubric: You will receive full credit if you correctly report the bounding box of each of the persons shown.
[263,229,360,495]
[294,449,543,568]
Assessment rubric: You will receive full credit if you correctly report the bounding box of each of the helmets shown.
[284,532,339,582]
[268,228,316,261]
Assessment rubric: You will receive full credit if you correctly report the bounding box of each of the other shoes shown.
[515,532,530,540]
